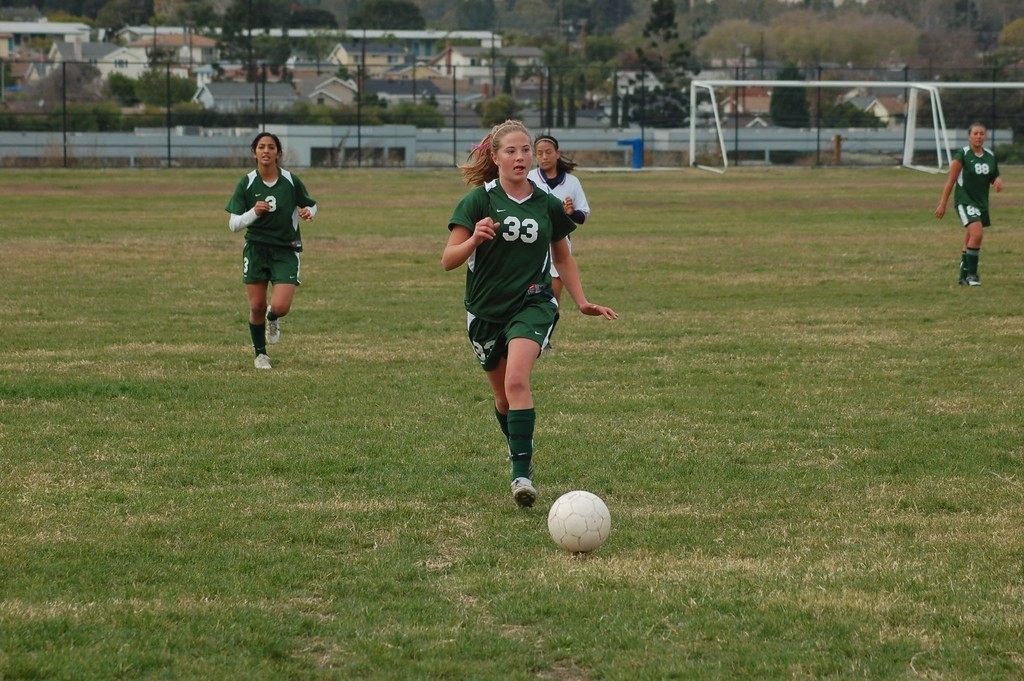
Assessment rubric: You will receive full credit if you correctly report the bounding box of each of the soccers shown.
[547,490,612,552]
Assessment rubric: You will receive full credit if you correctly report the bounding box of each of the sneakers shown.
[959,279,969,285]
[510,477,536,508]
[508,444,535,482]
[265,305,280,345]
[255,353,272,369]
[967,274,981,286]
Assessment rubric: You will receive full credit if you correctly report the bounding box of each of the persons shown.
[225,132,317,370]
[442,119,619,507]
[935,123,1003,286]
[527,137,590,350]
